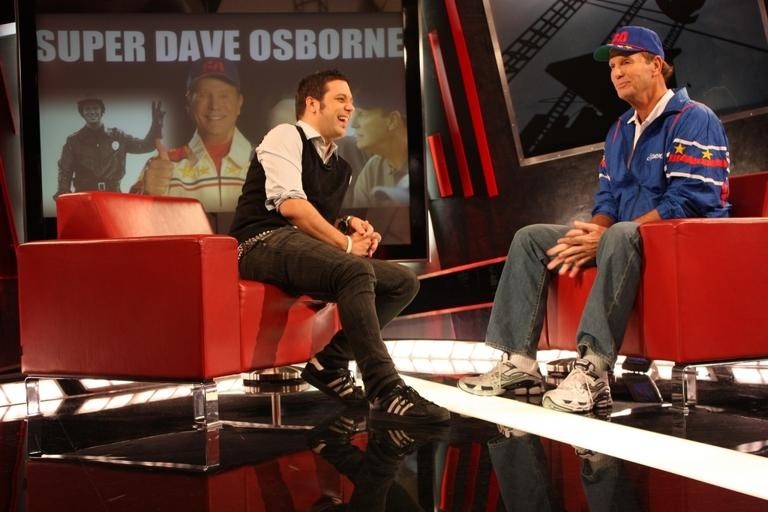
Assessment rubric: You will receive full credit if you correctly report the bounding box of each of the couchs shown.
[16,190,342,424]
[438,441,499,510]
[534,168,768,407]
[15,421,368,512]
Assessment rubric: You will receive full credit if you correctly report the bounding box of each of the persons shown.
[227,67,450,423]
[303,405,453,511]
[349,76,411,207]
[125,57,254,214]
[455,25,734,417]
[53,92,165,205]
[484,423,644,512]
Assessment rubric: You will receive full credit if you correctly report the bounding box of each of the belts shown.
[78,182,121,191]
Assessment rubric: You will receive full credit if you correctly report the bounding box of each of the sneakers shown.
[367,386,453,426]
[299,360,368,403]
[455,350,546,399]
[542,360,616,411]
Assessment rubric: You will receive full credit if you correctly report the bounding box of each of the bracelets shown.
[344,234,353,254]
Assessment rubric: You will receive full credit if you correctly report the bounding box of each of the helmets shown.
[78,92,105,113]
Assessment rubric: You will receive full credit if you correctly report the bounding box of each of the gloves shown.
[151,100,167,128]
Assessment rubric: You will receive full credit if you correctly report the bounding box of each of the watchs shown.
[337,215,354,237]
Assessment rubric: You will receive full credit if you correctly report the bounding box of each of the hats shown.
[354,61,406,113]
[186,57,240,91]
[594,23,666,62]
